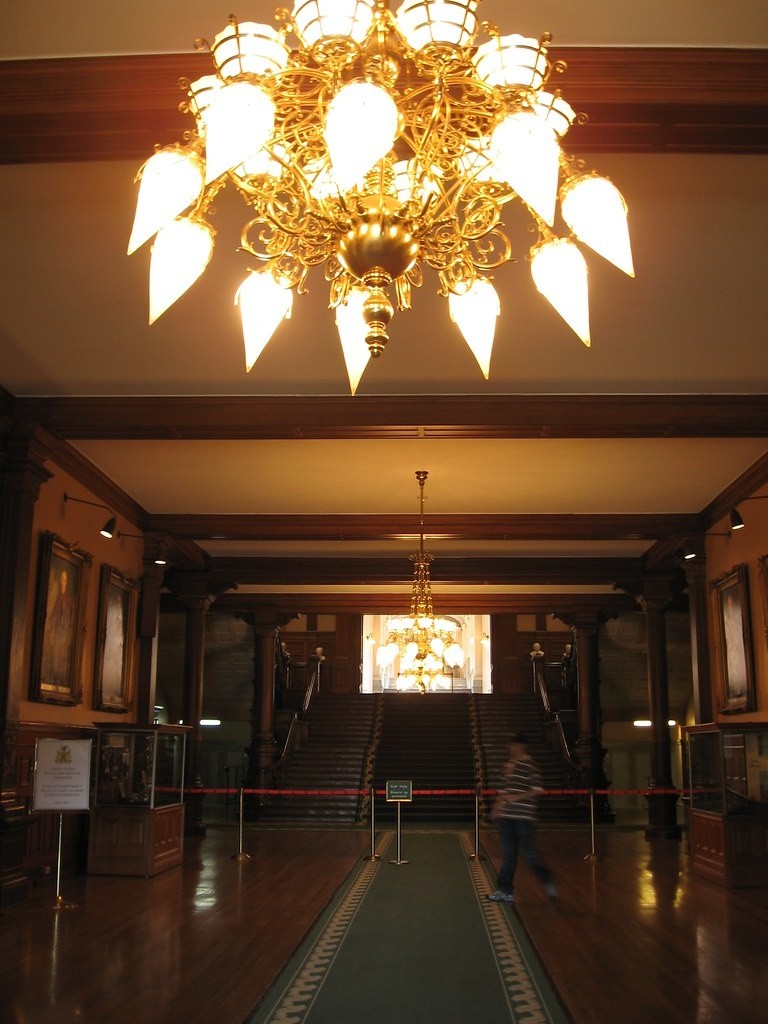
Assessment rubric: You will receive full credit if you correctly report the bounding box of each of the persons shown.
[486,732,560,903]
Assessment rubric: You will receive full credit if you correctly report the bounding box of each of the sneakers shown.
[486,890,512,902]
[545,883,558,899]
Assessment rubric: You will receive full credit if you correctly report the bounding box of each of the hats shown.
[503,732,528,743]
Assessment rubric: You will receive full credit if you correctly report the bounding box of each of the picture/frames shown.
[28,529,95,708]
[708,562,758,715]
[93,562,138,714]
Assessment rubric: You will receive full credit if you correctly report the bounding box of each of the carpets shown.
[246,828,569,1024]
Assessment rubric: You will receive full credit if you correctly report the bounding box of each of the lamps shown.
[730,495,768,529]
[126,0,634,398]
[117,530,168,564]
[366,633,375,645]
[480,633,489,644]
[376,471,466,696]
[682,532,731,559]
[63,492,117,539]
